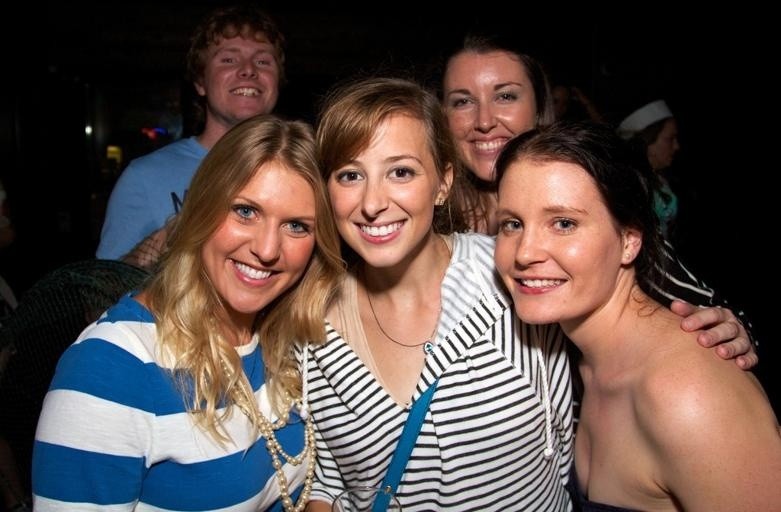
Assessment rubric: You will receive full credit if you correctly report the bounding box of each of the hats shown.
[609,95,675,143]
